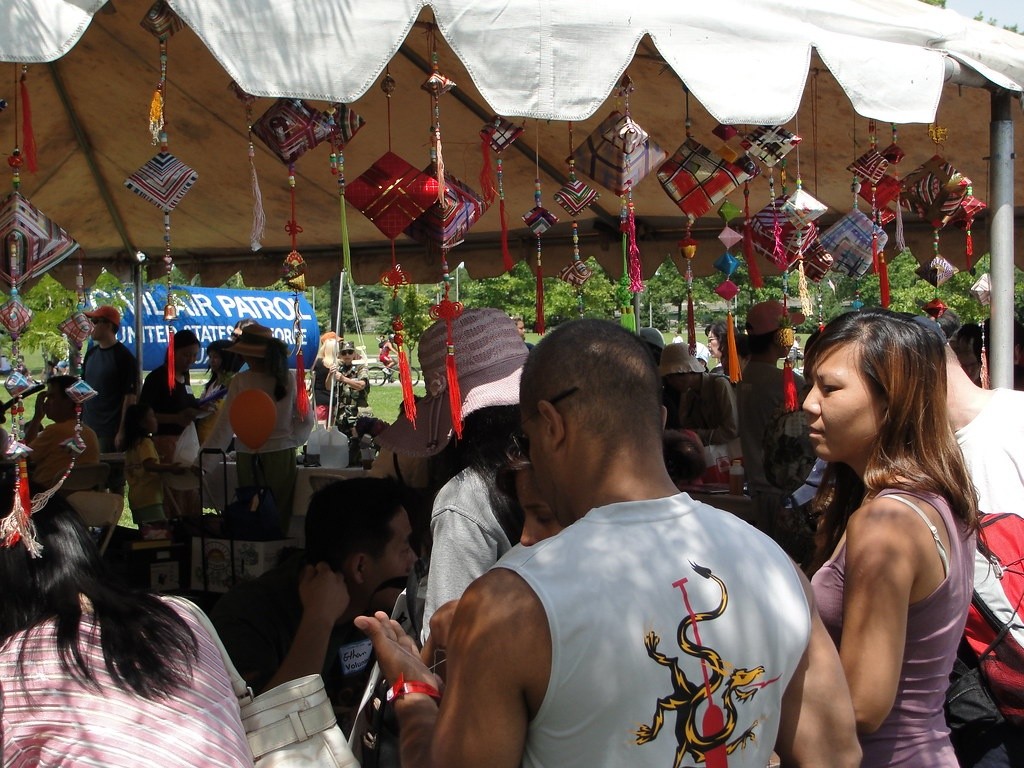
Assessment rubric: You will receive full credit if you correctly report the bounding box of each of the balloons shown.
[229,388,276,449]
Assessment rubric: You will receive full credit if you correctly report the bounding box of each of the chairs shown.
[51,462,125,557]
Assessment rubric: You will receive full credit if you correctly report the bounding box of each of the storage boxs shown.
[192,537,296,595]
[146,561,180,592]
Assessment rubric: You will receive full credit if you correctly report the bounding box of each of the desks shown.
[0,451,126,469]
[191,461,371,545]
[687,483,770,529]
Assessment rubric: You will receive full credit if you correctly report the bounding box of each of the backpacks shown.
[963,513,1024,724]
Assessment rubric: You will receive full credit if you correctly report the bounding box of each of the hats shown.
[340,340,354,351]
[640,327,665,349]
[374,306,528,458]
[84,306,120,328]
[221,322,273,358]
[661,343,706,376]
[747,301,804,335]
[321,332,345,344]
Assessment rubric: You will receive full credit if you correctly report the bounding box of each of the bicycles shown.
[368,356,421,387]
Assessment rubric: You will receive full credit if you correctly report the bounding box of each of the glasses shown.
[91,318,108,324]
[341,350,354,355]
[510,387,580,462]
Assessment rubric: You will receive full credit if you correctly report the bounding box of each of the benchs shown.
[778,347,804,368]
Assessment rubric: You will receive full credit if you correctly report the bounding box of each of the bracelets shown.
[385,673,443,702]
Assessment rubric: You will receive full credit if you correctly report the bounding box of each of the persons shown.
[0,301,1024,768]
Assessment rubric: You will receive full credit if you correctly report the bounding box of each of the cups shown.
[360,449,376,470]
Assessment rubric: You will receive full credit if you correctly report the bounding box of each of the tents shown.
[0,1,1024,389]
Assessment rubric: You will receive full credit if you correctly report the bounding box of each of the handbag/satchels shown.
[944,665,1004,761]
[177,594,362,768]
[169,420,201,489]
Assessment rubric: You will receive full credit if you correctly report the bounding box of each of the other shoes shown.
[382,367,391,375]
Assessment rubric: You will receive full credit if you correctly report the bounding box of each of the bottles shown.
[307,424,327,465]
[320,426,349,468]
[349,433,372,470]
[729,460,744,496]
